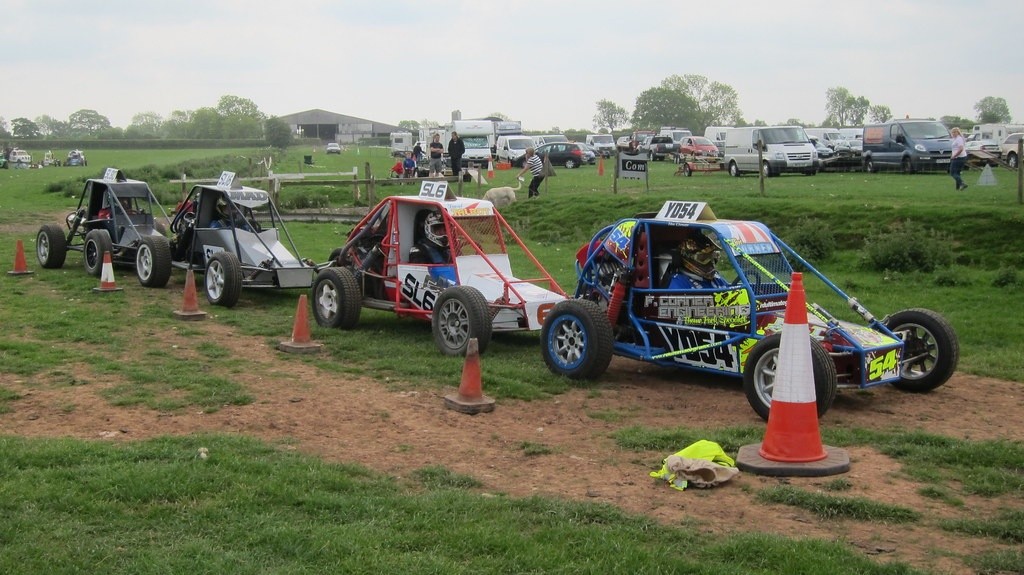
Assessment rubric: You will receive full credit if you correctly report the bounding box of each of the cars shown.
[325,142,342,155]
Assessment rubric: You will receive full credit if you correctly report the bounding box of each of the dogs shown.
[482,182,522,207]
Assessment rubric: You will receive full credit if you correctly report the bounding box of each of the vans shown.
[861,115,954,175]
[722,125,820,179]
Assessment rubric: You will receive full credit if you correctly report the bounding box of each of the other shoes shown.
[960,182,968,191]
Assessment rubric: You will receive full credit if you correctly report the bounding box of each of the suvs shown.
[512,142,582,169]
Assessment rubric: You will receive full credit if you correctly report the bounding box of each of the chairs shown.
[654,239,681,290]
[413,209,435,246]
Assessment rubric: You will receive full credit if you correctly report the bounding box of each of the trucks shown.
[805,128,865,172]
[585,134,617,159]
[616,124,735,165]
[389,120,597,169]
[961,121,1024,170]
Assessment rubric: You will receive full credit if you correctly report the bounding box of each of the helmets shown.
[214,193,240,221]
[107,191,129,207]
[425,212,453,251]
[677,233,723,279]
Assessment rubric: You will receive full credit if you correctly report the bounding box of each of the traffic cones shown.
[6,238,35,276]
[486,155,495,179]
[172,269,208,321]
[595,154,606,177]
[92,250,124,294]
[277,293,323,356]
[736,272,851,479]
[442,338,496,416]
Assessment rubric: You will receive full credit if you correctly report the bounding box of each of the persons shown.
[409,211,462,263]
[668,234,731,289]
[389,152,415,185]
[98,190,132,219]
[517,148,544,198]
[413,143,425,161]
[951,128,968,191]
[448,132,465,176]
[430,134,444,177]
[211,194,251,231]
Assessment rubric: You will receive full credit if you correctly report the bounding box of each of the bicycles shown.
[386,168,403,186]
[405,167,416,186]
